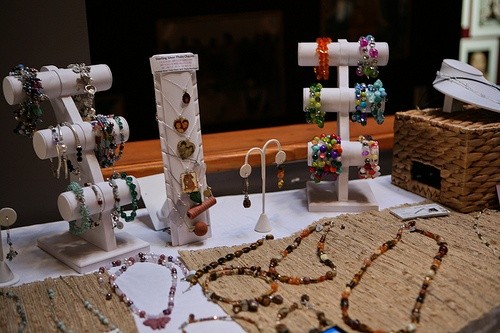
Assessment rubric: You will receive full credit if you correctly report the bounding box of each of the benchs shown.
[102,112,395,181]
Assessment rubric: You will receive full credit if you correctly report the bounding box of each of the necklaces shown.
[338,219,448,333]
[434,69,500,105]
[269,219,338,287]
[101,251,189,330]
[153,68,218,238]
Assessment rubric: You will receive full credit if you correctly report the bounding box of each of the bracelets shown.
[11,60,138,232]
[303,35,386,185]
[178,232,332,333]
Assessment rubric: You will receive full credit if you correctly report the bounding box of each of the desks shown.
[1,172,499,333]
